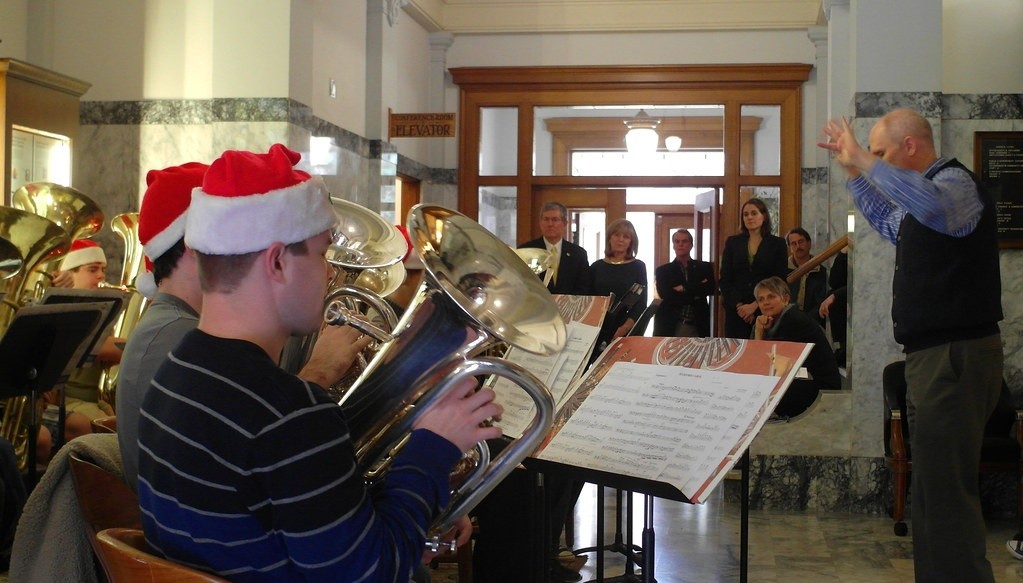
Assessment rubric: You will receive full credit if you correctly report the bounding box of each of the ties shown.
[550,246,558,286]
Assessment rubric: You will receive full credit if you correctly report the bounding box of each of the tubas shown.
[0,179,154,472]
[276,195,409,399]
[321,202,568,557]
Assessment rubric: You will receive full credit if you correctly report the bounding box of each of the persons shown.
[818,109,1005,583]
[1,144,853,583]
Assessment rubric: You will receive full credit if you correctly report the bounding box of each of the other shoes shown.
[554,564,582,583]
[556,547,575,561]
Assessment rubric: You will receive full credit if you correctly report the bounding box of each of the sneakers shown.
[1006,540,1023,559]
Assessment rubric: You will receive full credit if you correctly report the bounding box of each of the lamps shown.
[665,135,682,152]
[622,107,661,160]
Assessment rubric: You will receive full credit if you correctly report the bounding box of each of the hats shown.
[59,238,107,271]
[136,161,212,261]
[184,143,337,255]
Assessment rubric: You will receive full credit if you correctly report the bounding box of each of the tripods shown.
[567,284,664,583]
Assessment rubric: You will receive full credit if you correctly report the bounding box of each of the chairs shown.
[882,361,1023,536]
[65,412,235,583]
